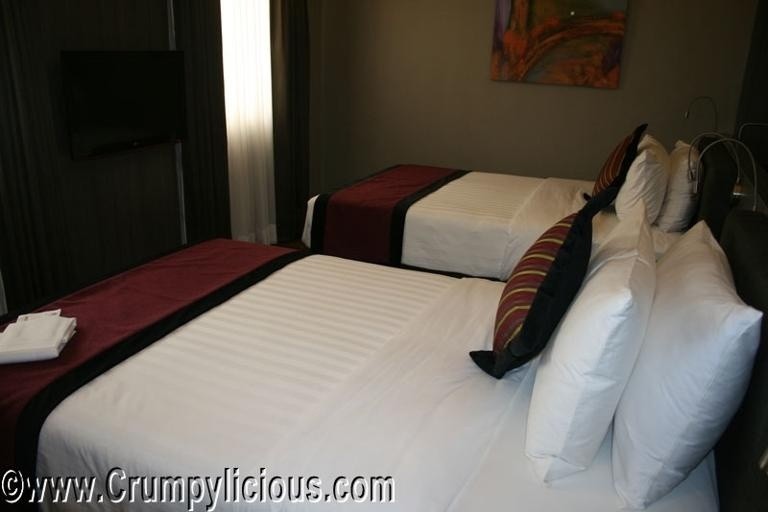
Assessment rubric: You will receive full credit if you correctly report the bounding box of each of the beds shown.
[0,211,768,512]
[302,133,740,283]
